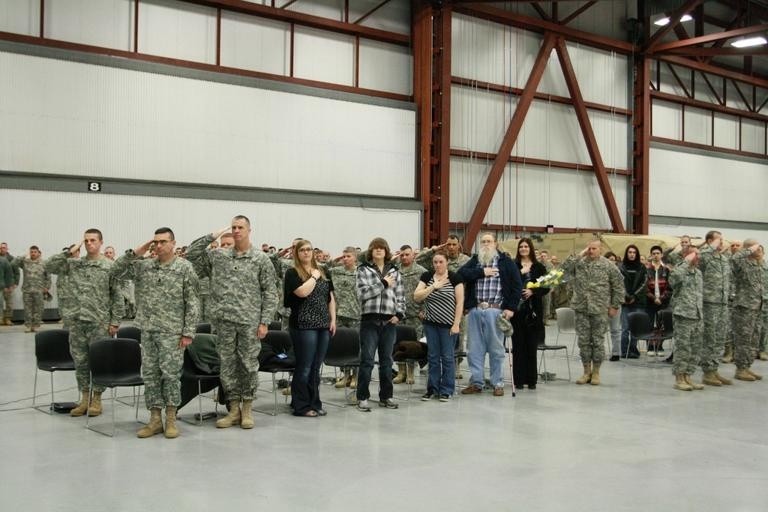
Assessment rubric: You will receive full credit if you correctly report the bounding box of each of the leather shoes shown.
[516,384,523,388]
[317,409,327,415]
[609,356,619,361]
[622,352,638,358]
[528,384,536,389]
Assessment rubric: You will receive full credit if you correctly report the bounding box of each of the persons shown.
[0,256,14,325]
[41,228,126,417]
[645,245,673,357]
[0,242,21,325]
[662,235,692,363]
[10,245,52,333]
[456,231,523,396]
[176,247,182,254]
[413,252,465,402]
[536,251,555,326]
[604,250,623,362]
[548,255,557,321]
[414,234,471,379]
[103,245,115,261]
[752,245,768,361]
[283,240,337,418]
[639,255,646,264]
[218,232,235,250]
[721,239,743,364]
[210,241,219,249]
[729,238,763,382]
[269,237,303,395]
[559,237,626,386]
[511,237,552,390]
[619,245,649,359]
[667,247,705,392]
[534,248,539,257]
[315,250,323,262]
[355,238,407,412]
[391,244,428,385]
[698,230,734,386]
[183,215,279,429]
[323,245,362,389]
[109,227,202,439]
[138,245,157,261]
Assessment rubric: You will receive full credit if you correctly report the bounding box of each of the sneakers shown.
[356,399,371,412]
[439,393,452,401]
[494,387,504,395]
[647,351,654,356]
[658,351,664,356]
[379,398,399,409]
[462,385,481,393]
[421,390,434,400]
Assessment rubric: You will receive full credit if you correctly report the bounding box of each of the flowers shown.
[517,268,567,309]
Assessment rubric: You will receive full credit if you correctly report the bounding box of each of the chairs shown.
[554,309,580,357]
[113,328,142,406]
[390,325,429,402]
[319,329,379,407]
[501,333,514,384]
[255,321,283,391]
[195,324,219,401]
[625,312,673,368]
[656,310,674,357]
[85,339,149,438]
[538,322,570,385]
[454,335,468,396]
[251,330,297,417]
[32,329,80,416]
[174,334,226,426]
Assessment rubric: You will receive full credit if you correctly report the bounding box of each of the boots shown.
[87,392,103,416]
[758,351,768,360]
[70,390,89,417]
[745,368,762,380]
[590,362,601,385]
[672,374,693,391]
[215,399,241,428]
[31,326,39,332]
[576,361,591,384]
[735,368,755,381]
[136,407,163,438]
[713,370,732,385]
[282,385,291,395]
[5,319,14,326]
[164,407,179,438]
[406,362,415,384]
[240,400,254,429]
[721,344,734,363]
[350,374,358,388]
[334,370,351,388]
[684,374,704,389]
[393,364,406,384]
[702,371,722,385]
[25,326,32,332]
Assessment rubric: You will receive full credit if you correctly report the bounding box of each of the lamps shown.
[731,37,766,49]
[654,14,693,27]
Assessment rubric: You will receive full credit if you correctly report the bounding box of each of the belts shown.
[476,302,499,309]
